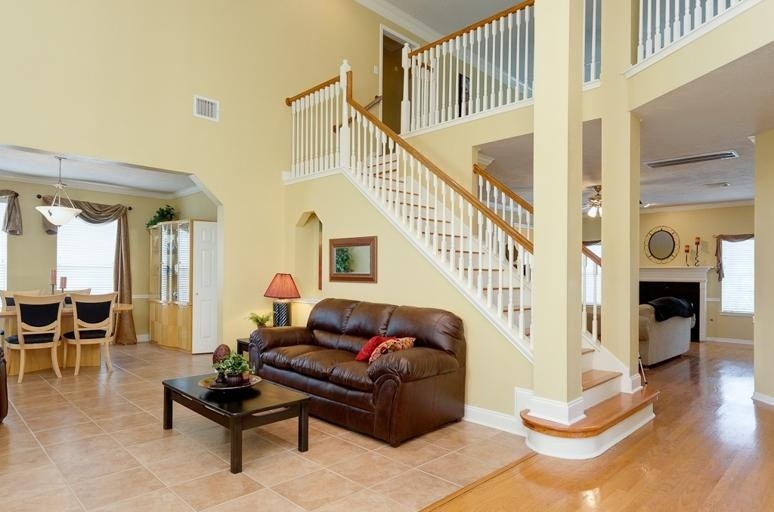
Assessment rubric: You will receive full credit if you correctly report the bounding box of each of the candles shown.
[49,268,57,284]
[684,244,690,253]
[695,237,701,245]
[59,277,67,288]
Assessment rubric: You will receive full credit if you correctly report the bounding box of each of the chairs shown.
[60,292,119,373]
[55,289,92,306]
[4,302,64,383]
[0,289,45,310]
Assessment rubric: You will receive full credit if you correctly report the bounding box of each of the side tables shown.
[236,336,250,358]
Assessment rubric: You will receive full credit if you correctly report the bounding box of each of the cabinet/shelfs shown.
[147,219,217,354]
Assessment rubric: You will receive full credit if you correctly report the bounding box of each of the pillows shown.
[354,336,396,361]
[368,337,416,364]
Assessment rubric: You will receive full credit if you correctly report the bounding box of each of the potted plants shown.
[250,310,275,330]
[214,353,250,387]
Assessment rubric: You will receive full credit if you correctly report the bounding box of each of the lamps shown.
[34,158,82,225]
[263,273,301,325]
[586,185,602,217]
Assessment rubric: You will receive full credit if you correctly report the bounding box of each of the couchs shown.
[249,298,467,448]
[639,299,697,366]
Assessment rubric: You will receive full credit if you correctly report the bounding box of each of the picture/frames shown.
[458,73,469,117]
[328,236,378,283]
